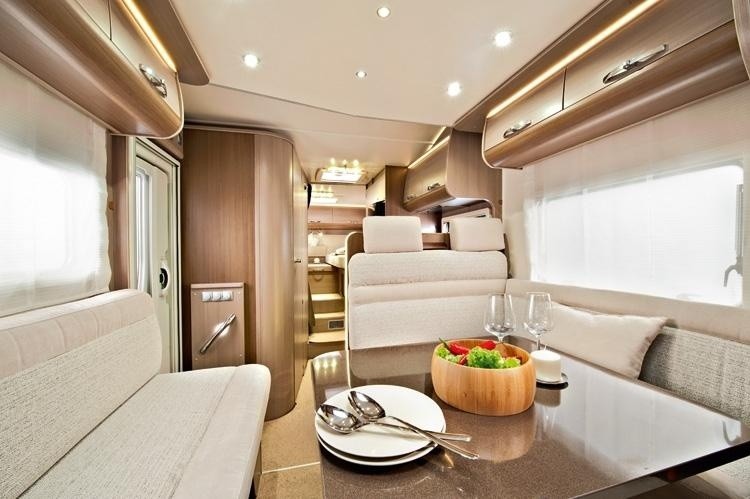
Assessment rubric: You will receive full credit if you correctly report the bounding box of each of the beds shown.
[325,254,345,295]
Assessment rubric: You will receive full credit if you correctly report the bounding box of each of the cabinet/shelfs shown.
[109,0,182,120]
[481,59,564,163]
[402,136,456,213]
[563,1,737,110]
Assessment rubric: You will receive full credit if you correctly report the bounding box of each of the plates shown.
[536,373,569,387]
[313,384,446,467]
[320,454,438,490]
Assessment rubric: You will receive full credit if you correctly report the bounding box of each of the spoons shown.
[315,400,472,443]
[348,390,479,461]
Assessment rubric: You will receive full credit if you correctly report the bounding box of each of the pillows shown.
[449,217,505,251]
[362,216,423,253]
[534,300,668,381]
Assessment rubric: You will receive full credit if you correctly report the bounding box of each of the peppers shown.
[458,341,506,364]
[438,336,470,355]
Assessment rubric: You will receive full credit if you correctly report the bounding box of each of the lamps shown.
[315,158,368,184]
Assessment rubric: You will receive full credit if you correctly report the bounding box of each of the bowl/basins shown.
[442,402,538,462]
[431,338,537,417]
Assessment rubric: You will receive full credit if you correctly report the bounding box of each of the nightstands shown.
[308,263,332,294]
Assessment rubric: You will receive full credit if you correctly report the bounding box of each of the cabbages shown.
[467,345,521,369]
[437,346,464,364]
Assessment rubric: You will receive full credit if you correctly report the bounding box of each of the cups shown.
[484,293,517,344]
[523,291,556,349]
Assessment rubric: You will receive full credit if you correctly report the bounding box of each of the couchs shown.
[345,251,749,499]
[0,286,272,499]
